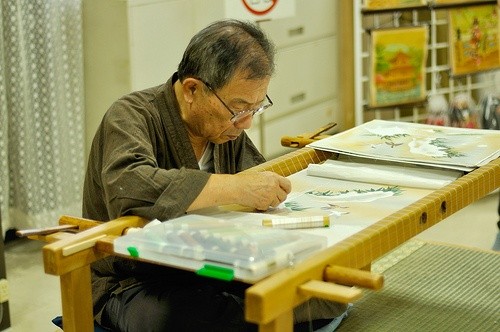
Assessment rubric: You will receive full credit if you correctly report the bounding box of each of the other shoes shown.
[294,309,347,332]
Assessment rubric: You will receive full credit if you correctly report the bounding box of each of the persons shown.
[82,18,350,332]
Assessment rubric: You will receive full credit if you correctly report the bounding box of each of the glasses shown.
[185,76,274,122]
